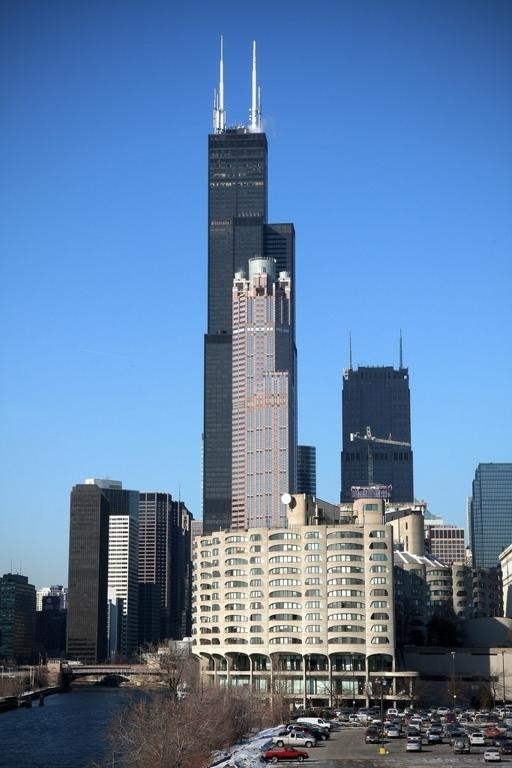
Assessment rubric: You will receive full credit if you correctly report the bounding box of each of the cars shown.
[261,707,332,761]
[334,704,512,761]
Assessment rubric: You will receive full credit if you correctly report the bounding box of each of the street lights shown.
[374,676,388,747]
[451,652,456,709]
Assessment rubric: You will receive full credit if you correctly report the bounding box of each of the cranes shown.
[351,425,411,499]
